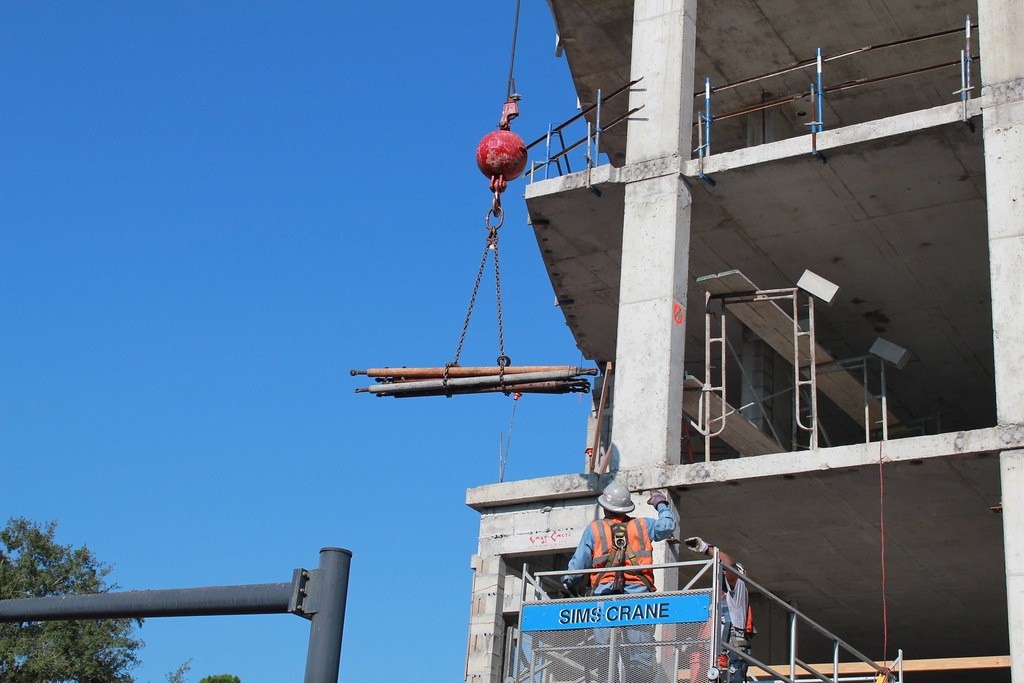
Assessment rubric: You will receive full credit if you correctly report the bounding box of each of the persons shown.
[683,537,757,683]
[562,479,675,682]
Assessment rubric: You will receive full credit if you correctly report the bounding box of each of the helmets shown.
[597,482,635,513]
[736,561,746,575]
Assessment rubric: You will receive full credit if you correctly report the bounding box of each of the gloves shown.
[646,489,670,511]
[684,536,714,555]
